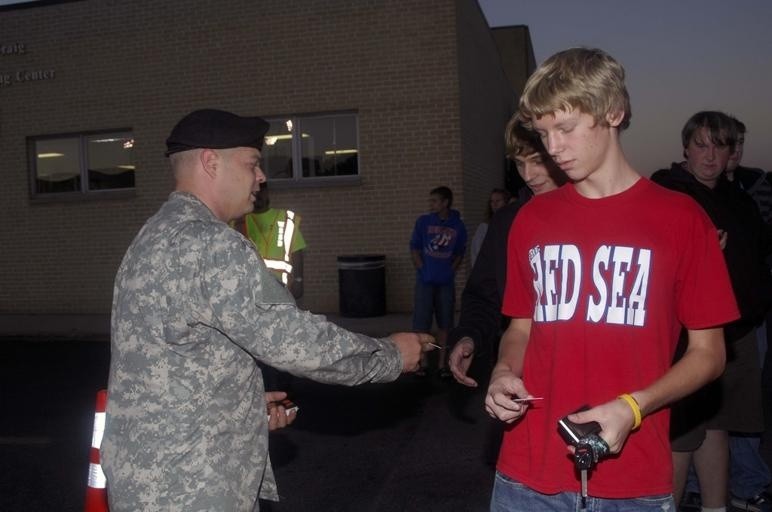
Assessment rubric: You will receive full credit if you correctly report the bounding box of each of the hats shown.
[165,109,270,157]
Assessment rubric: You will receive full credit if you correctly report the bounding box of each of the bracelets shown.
[293,276,304,282]
[618,393,643,430]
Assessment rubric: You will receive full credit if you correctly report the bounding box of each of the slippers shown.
[415,367,450,378]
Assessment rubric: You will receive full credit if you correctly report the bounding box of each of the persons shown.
[483,45,744,511]
[97,104,437,511]
[647,108,768,512]
[409,185,468,380]
[229,182,308,302]
[675,116,771,512]
[447,105,567,470]
[467,189,510,275]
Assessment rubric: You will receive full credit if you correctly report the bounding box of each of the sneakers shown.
[730,491,772,512]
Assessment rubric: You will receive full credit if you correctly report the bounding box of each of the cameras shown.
[558,405,604,445]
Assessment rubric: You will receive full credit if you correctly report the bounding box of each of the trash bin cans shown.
[337,254,387,319]
[288,249,305,299]
